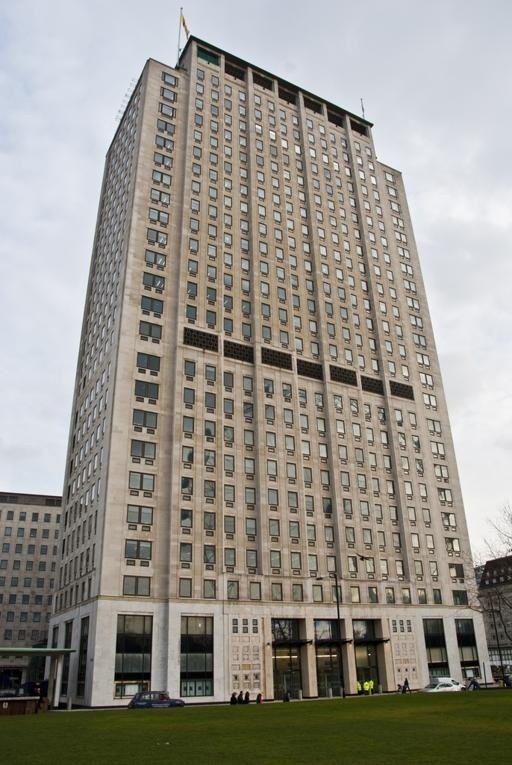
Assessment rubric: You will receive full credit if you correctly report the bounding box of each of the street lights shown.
[316,573,346,699]
[477,591,506,690]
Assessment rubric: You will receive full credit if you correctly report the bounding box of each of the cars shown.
[419,677,467,692]
[128,690,185,708]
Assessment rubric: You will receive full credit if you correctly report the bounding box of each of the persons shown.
[286,690,291,702]
[34,699,42,713]
[245,691,249,703]
[363,679,369,695]
[357,680,362,695]
[238,691,243,704]
[368,679,373,694]
[256,692,263,704]
[506,665,511,673]
[403,677,411,693]
[230,692,237,704]
